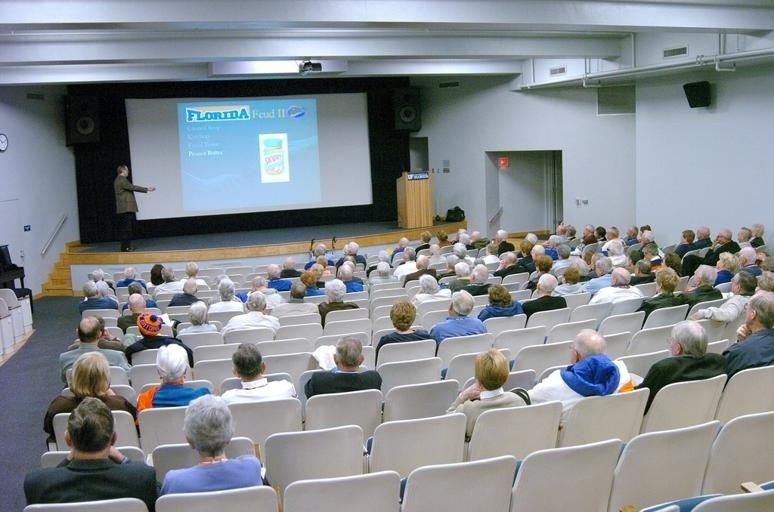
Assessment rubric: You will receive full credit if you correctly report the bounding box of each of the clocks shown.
[0,134,8,152]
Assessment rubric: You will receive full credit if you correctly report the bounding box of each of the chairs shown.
[23,239,774,512]
[0,288,25,343]
[0,298,14,355]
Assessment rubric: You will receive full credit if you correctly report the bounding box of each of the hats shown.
[136,311,165,336]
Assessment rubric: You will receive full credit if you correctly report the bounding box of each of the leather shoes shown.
[120,247,135,252]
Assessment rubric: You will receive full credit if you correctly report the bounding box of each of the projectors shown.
[298,63,322,73]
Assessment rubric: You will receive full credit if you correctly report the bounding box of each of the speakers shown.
[64,95,101,146]
[393,86,421,131]
[683,81,711,108]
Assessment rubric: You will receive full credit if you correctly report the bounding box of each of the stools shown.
[13,288,34,314]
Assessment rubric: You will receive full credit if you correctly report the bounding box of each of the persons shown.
[24,393,263,510]
[23,223,773,442]
[114,164,155,252]
